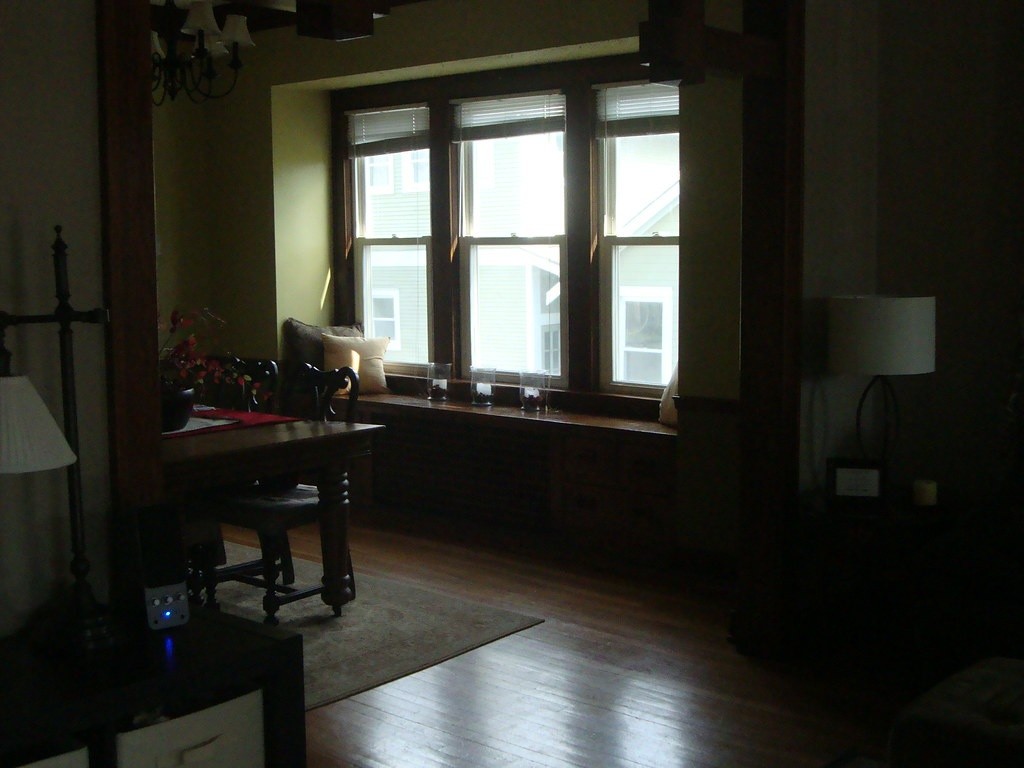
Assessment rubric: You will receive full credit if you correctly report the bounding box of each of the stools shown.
[888,656,1024,767]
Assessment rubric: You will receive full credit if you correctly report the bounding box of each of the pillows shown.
[284,318,362,373]
[658,361,679,428]
[321,332,392,396]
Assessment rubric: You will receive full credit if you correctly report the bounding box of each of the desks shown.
[798,486,952,679]
[157,400,388,617]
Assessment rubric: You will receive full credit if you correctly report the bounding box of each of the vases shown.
[159,375,195,432]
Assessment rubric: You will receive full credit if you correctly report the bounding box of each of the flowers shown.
[156,302,273,406]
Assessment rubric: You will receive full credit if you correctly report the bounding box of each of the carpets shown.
[188,538,545,713]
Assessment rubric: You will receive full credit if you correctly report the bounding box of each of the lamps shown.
[148,0,257,106]
[0,345,78,473]
[828,292,934,503]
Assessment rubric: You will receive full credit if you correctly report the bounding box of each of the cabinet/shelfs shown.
[0,598,304,767]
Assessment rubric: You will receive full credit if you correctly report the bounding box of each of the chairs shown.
[193,359,357,625]
